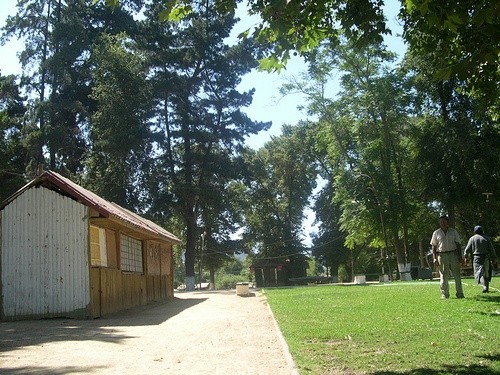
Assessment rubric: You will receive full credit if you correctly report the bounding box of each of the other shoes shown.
[456,293,465,299]
[482,288,488,293]
[441,294,449,298]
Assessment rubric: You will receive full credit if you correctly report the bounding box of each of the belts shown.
[436,250,457,253]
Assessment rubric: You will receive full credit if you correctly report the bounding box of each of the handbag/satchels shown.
[455,241,461,255]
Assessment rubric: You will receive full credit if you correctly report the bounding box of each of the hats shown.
[440,215,448,219]
[474,225,483,233]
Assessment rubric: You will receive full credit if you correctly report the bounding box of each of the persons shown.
[429,216,466,298]
[463,225,499,293]
